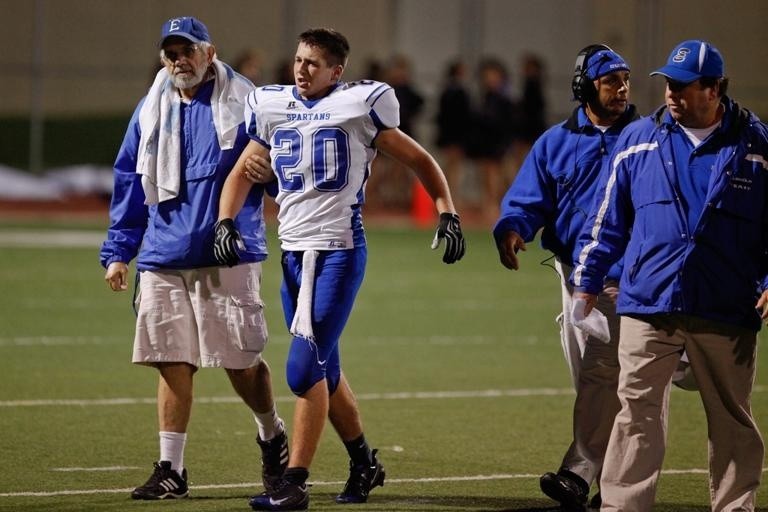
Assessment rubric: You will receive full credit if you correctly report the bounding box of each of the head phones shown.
[571,43,612,105]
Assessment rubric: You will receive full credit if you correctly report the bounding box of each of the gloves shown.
[430,212,465,264]
[212,219,243,268]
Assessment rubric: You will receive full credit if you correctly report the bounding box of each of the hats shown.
[586,50,631,80]
[158,16,211,49]
[650,40,725,84]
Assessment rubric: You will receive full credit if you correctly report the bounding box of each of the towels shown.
[136,58,256,206]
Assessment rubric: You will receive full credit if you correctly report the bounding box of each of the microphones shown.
[575,55,610,92]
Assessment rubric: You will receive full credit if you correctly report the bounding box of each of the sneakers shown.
[248,481,310,511]
[256,419,290,490]
[132,460,189,500]
[539,472,589,509]
[335,450,384,504]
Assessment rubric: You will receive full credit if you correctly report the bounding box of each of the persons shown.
[491,45,647,505]
[233,49,551,226]
[212,28,466,511]
[570,41,767,511]
[97,15,290,501]
